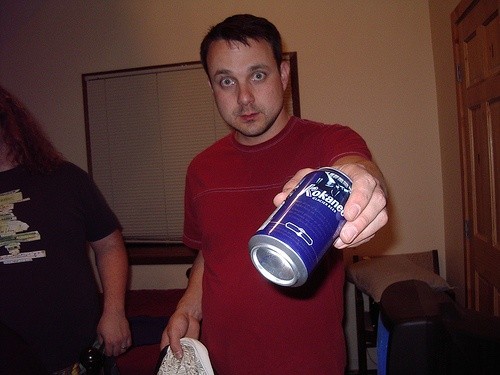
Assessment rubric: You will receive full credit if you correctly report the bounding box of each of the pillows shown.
[345,255,458,305]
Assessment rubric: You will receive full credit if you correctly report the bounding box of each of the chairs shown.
[353,250,456,375]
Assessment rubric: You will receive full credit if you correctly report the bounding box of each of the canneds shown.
[248,166,352,287]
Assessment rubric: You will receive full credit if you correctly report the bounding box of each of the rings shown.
[119,343,128,352]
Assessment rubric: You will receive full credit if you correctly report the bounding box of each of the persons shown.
[161,13,389,374]
[0,84,133,375]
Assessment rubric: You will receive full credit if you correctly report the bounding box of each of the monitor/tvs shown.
[377,279,500,375]
